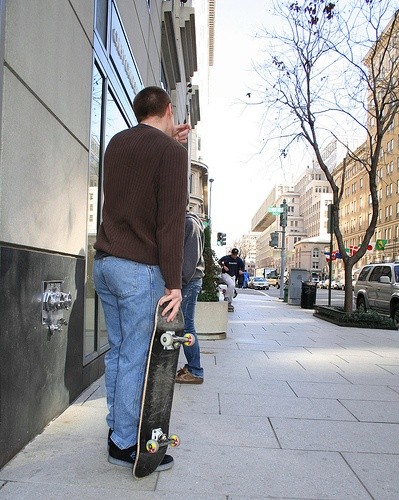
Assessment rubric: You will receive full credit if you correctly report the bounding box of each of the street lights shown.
[209,179,214,220]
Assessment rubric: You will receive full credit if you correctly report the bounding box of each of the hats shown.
[232,248,239,254]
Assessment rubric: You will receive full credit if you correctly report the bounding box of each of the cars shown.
[248,277,269,290]
[266,275,280,289]
[317,279,356,291]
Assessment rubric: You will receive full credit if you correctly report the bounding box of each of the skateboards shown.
[133,294,195,480]
[228,305,234,312]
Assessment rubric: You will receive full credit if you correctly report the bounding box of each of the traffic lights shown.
[217,233,226,247]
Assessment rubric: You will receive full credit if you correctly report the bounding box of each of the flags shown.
[374,239,386,250]
[324,243,363,262]
[365,242,374,254]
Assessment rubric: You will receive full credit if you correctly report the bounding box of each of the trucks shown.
[254,267,277,278]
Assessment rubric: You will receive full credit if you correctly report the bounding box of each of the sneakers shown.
[107,428,116,450]
[108,444,175,472]
[175,367,204,384]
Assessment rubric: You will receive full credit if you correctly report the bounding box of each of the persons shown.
[92,86,191,473]
[235,269,250,289]
[175,187,206,385]
[218,248,244,311]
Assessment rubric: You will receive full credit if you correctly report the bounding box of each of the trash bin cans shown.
[300,281,319,308]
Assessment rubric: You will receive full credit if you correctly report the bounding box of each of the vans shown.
[354,263,399,329]
